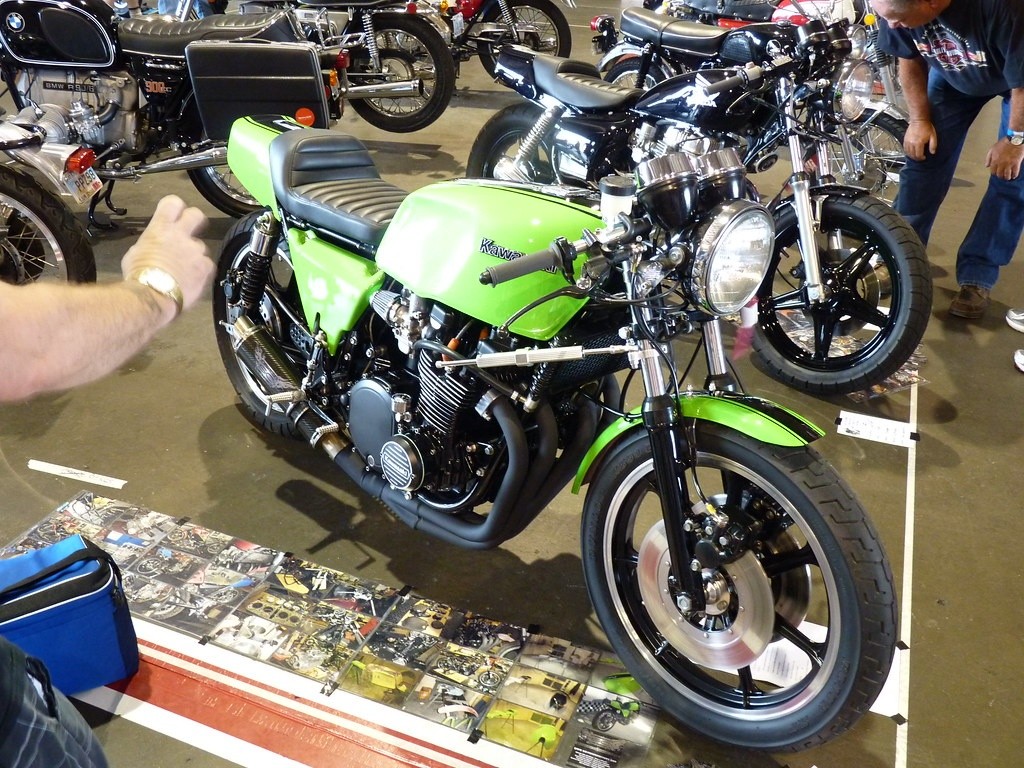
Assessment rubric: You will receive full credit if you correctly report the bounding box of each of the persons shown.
[873,0,1024,373]
[0,193,217,768]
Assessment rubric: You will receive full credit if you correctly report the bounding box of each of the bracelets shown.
[1007,128,1024,137]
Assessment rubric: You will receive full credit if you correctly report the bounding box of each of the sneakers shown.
[861,261,892,294]
[949,285,990,318]
[1014,349,1024,372]
[1006,309,1024,333]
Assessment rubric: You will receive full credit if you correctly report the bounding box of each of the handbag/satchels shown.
[0,534,139,697]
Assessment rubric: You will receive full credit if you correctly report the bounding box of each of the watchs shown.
[1006,135,1024,146]
[125,265,184,321]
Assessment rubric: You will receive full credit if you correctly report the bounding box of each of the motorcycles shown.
[211,113,897,754]
[466,1,934,396]
[0,0,579,287]
